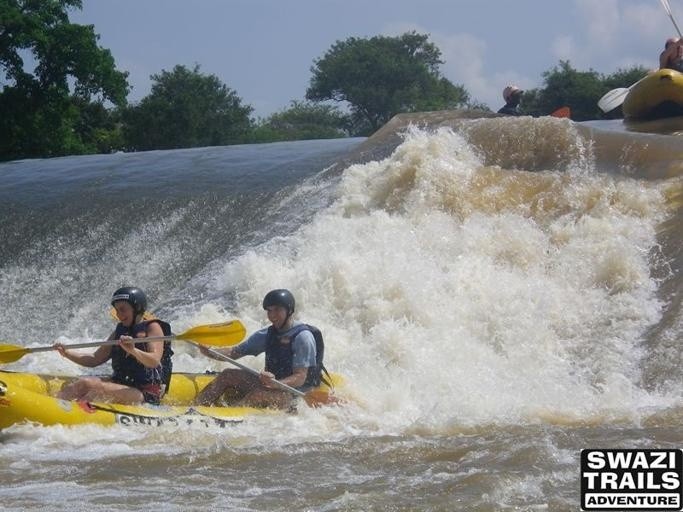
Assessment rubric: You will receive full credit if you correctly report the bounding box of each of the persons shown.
[498,86,524,115]
[660,37,683,71]
[191,290,325,408]
[54,287,174,406]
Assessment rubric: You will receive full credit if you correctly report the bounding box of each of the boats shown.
[0,367,349,435]
[622,65,683,118]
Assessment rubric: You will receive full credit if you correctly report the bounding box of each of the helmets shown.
[111,286,148,314]
[503,85,523,100]
[665,37,679,49]
[263,289,295,310]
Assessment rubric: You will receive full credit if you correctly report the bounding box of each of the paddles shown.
[597,68,660,114]
[171,331,347,408]
[0,319,245,364]
[661,0,682,38]
